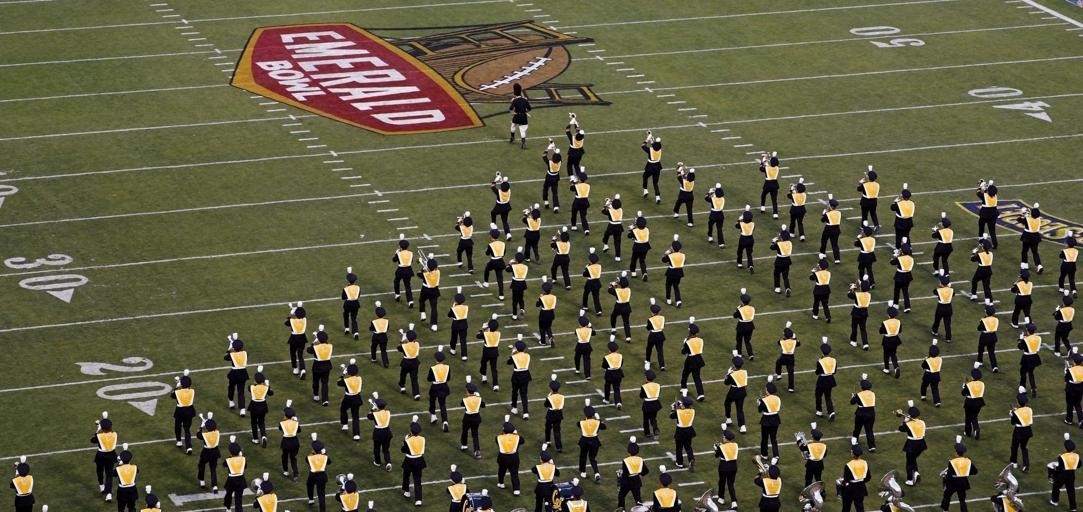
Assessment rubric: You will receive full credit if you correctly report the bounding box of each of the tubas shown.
[994,464,1014,507]
[752,455,768,478]
[876,469,918,512]
[696,489,720,512]
[799,481,827,512]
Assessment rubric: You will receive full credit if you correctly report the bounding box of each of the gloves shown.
[647,133,654,142]
[549,142,556,150]
[569,118,578,125]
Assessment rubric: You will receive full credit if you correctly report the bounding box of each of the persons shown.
[288,117,1082,510]
[113,451,139,512]
[223,442,247,512]
[197,419,221,493]
[170,376,196,455]
[223,340,249,417]
[248,373,274,447]
[252,480,278,512]
[140,494,162,512]
[90,419,117,501]
[279,408,301,480]
[509,92,531,148]
[10,462,35,512]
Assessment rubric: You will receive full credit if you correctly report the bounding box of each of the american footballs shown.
[416,35,571,100]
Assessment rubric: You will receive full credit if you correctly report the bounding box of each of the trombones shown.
[892,408,921,424]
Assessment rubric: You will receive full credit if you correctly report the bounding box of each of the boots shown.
[510,132,514,143]
[522,138,525,149]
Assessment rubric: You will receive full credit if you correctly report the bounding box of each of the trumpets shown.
[496,171,503,182]
[568,111,581,131]
[548,137,557,152]
[339,364,346,371]
[678,161,688,178]
[367,399,378,410]
[508,344,517,352]
[646,130,655,146]
[312,330,320,341]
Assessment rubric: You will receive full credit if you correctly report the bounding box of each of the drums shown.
[460,494,491,512]
[549,481,578,511]
[630,505,650,512]
[1046,461,1061,484]
[642,501,654,510]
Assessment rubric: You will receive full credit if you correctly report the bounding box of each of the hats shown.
[17,463,30,476]
[514,83,521,95]
[577,132,584,141]
[653,141,662,151]
[552,153,562,163]
